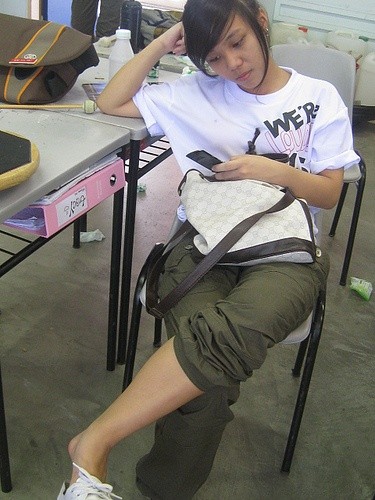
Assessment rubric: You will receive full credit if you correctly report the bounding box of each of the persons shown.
[57,0,361,500]
[70,0,126,42]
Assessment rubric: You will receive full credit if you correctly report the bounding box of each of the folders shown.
[3,159,125,238]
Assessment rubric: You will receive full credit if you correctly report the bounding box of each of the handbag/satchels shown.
[145,169,316,319]
[0,13,100,104]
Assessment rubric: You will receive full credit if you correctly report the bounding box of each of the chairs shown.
[270,44,367,286]
[120,202,329,480]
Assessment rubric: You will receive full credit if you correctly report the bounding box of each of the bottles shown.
[120,0,142,54]
[109,29,134,81]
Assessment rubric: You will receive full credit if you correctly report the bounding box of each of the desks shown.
[0,33,211,492]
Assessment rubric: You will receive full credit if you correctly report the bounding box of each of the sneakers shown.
[58,463,122,500]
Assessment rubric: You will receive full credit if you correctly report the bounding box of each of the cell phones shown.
[186,150,224,172]
